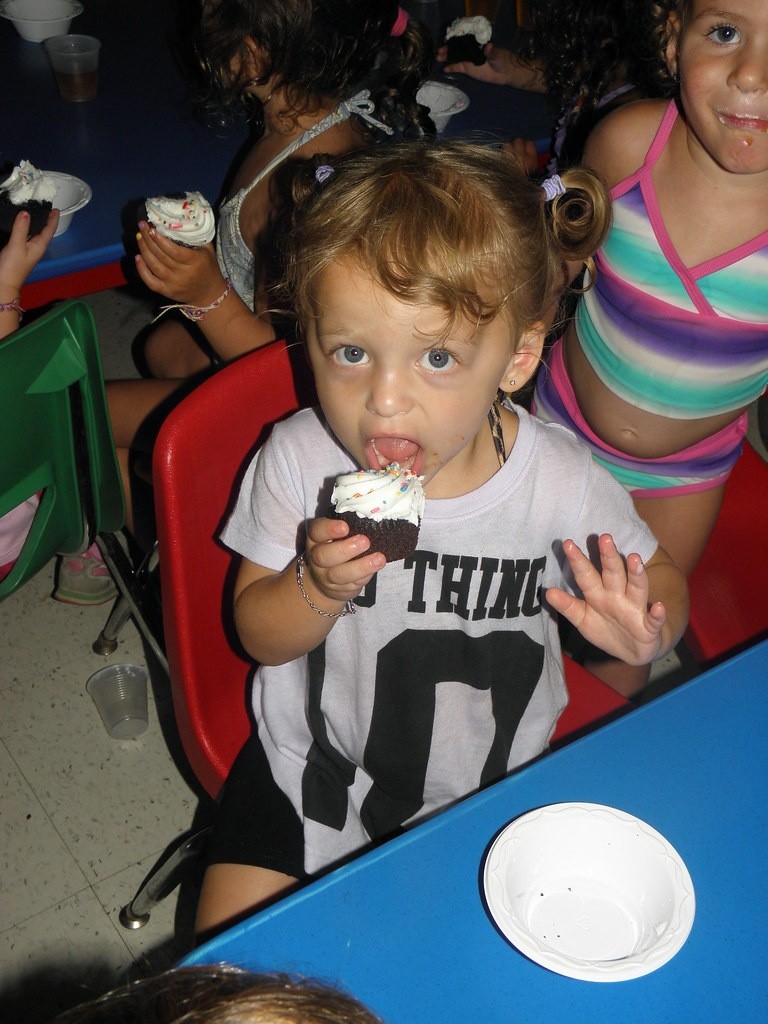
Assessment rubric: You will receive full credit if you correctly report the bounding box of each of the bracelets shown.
[295,552,357,619]
[0,298,25,321]
[150,278,233,324]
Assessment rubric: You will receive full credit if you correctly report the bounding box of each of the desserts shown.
[144,190,216,248]
[0,159,57,238]
[330,462,425,563]
[445,15,492,66]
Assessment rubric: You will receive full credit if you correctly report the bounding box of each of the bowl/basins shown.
[415,79,470,136]
[0,0,84,43]
[10,170,93,238]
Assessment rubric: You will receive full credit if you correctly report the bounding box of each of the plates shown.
[484,801,695,981]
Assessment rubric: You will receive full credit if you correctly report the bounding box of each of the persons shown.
[54,955,385,1024]
[439,1,768,698]
[1,0,436,604]
[196,134,688,951]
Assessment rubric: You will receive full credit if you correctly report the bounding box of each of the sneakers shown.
[55,551,119,607]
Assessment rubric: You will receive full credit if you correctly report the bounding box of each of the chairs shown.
[0,137,768,931]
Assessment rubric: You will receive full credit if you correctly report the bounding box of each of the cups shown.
[45,34,102,103]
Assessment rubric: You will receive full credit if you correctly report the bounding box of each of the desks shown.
[0,0,599,315]
[172,638,768,1024]
